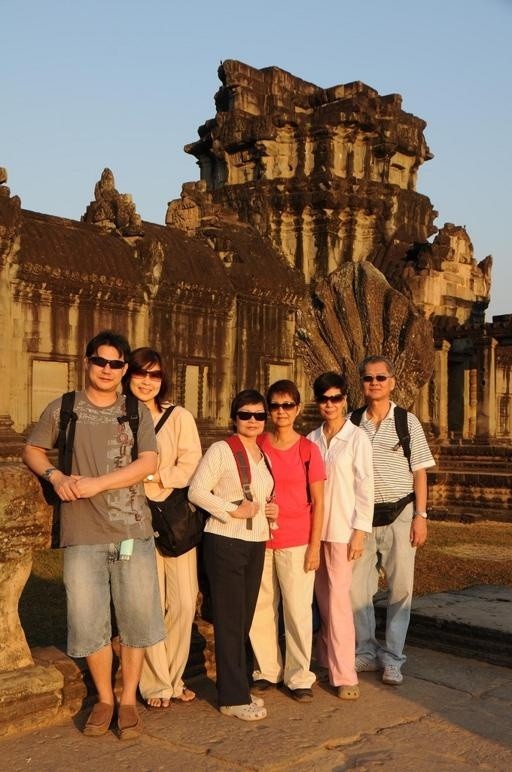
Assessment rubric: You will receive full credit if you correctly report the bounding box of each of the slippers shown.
[143,688,196,712]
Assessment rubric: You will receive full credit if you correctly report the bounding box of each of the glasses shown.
[131,369,162,380]
[90,357,124,369]
[239,411,266,421]
[270,403,295,411]
[362,376,387,382]
[317,395,344,403]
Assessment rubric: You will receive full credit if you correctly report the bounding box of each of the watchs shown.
[146,473,154,482]
[40,467,57,481]
[414,510,429,519]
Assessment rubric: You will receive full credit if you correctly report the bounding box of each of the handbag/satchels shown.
[149,486,211,557]
[373,502,397,527]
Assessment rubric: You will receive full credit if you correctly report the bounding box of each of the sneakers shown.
[219,653,404,722]
[82,703,144,741]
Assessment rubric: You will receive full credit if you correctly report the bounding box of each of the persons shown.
[256,379,328,704]
[118,346,204,714]
[22,328,166,744]
[306,370,375,702]
[346,356,437,687]
[186,388,280,723]
